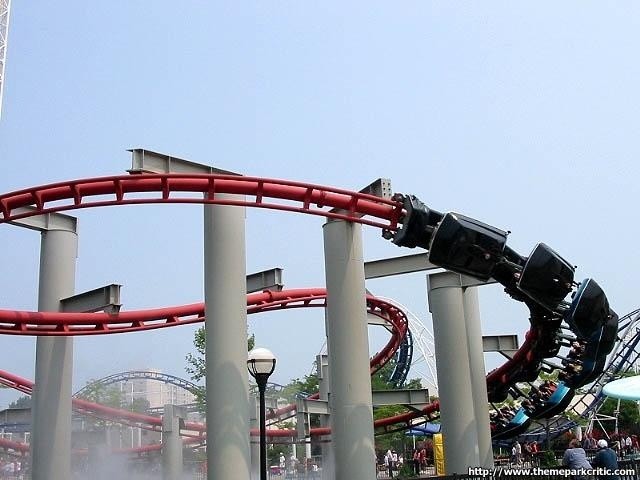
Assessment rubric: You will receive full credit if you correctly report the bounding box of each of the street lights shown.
[246,348,277,480]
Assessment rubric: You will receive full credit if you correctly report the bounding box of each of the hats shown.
[597,439,608,448]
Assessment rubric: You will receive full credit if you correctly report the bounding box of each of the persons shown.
[562,438,596,480]
[592,439,623,480]
[580,435,598,458]
[388,446,394,477]
[613,432,640,461]
[413,447,427,476]
[280,448,314,479]
[488,405,517,431]
[521,341,587,414]
[513,272,521,281]
[509,439,540,468]
